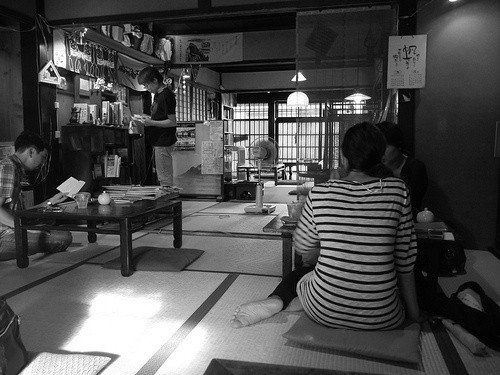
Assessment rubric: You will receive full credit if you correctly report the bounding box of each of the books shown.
[56,177,86,199]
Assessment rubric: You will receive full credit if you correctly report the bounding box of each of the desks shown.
[14,197,183,277]
[263,170,464,289]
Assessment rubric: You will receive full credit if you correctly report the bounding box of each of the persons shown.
[232,121,422,330]
[378,121,428,220]
[0,131,73,262]
[133,67,179,187]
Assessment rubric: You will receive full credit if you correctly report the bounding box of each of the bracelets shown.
[47,200,52,205]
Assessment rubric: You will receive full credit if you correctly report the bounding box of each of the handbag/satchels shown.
[0,299,27,375]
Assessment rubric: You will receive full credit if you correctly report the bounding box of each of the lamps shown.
[343,63,371,104]
[287,70,309,106]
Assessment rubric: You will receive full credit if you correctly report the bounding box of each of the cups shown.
[72,193,90,209]
[287,202,302,219]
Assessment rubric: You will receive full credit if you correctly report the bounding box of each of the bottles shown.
[98,205,110,214]
[98,190,110,205]
[417,208,433,222]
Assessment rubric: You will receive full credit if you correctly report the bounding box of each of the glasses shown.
[144,82,151,90]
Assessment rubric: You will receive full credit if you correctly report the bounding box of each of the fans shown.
[243,136,282,214]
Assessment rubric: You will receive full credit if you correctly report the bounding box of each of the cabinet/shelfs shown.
[222,104,235,147]
[60,125,155,194]
